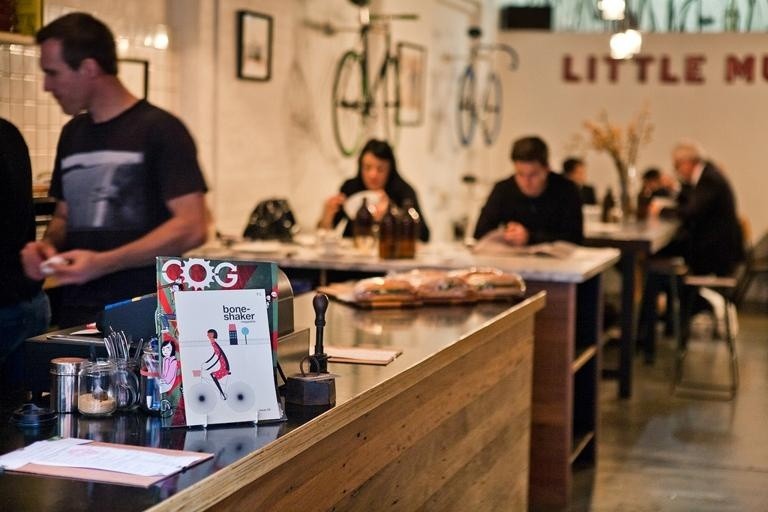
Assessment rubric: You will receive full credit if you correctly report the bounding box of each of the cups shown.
[77,359,136,415]
[117,361,143,410]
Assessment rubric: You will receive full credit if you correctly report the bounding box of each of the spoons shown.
[104,326,143,407]
[89,342,108,401]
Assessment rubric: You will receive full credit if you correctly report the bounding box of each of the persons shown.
[648,136,754,276]
[20,12,208,325]
[637,168,679,220]
[562,158,597,203]
[472,136,584,248]
[314,140,430,244]
[0,112,53,339]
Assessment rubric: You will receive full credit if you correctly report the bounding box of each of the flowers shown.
[563,101,664,192]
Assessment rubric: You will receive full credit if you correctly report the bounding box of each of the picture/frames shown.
[237,11,274,82]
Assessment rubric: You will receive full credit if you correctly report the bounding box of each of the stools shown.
[674,279,742,404]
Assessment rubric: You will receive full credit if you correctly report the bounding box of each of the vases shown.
[619,195,634,225]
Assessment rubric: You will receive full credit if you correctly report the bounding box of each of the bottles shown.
[352,197,373,246]
[636,182,652,221]
[602,187,616,221]
[395,200,416,258]
[378,204,396,259]
[77,361,119,417]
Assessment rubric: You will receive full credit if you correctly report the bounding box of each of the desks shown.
[184,229,621,512]
[582,204,687,401]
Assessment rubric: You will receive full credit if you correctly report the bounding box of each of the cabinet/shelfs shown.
[0,286,547,512]
